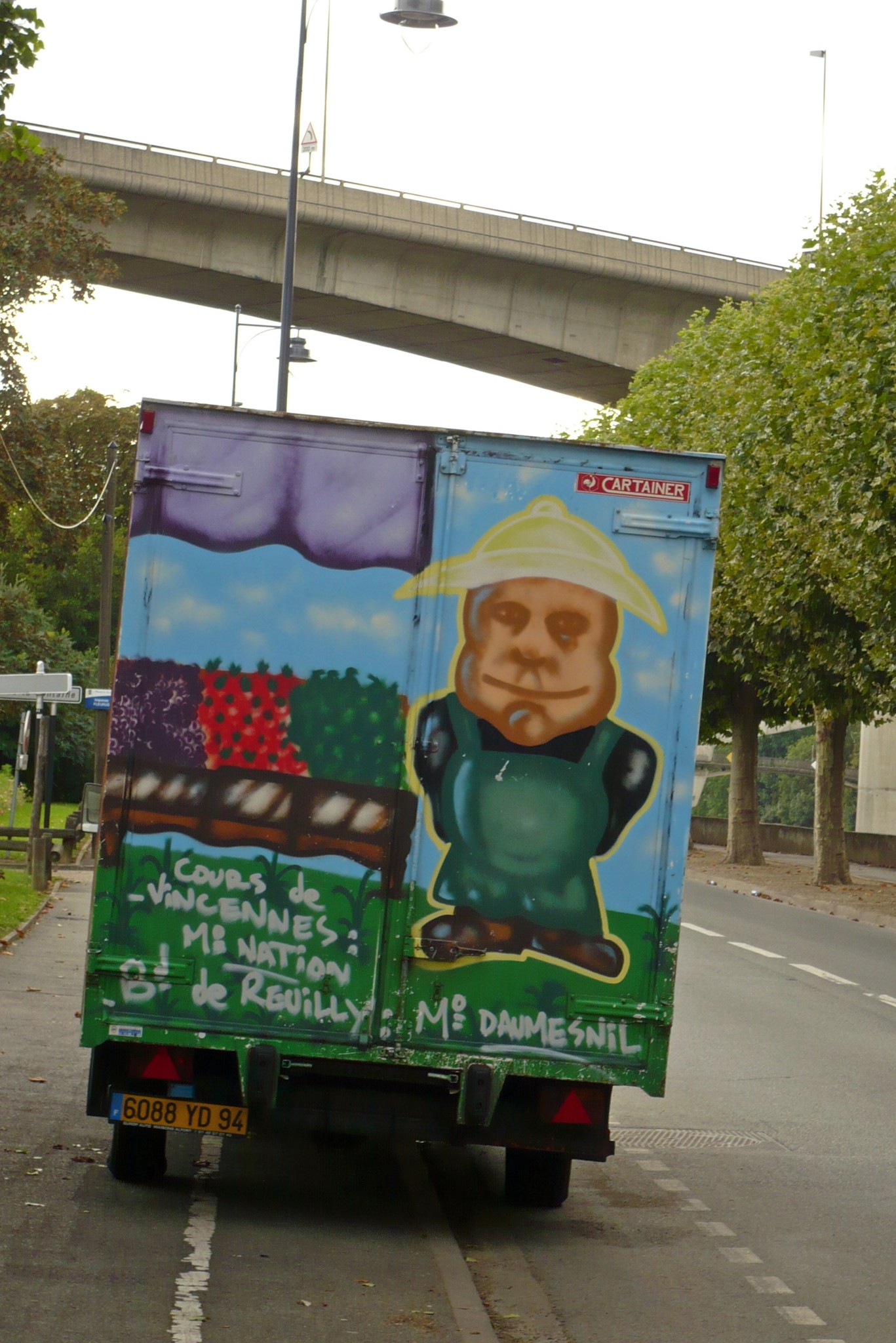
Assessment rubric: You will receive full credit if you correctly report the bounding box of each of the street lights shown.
[228,305,318,407]
[276,0,459,416]
[807,49,828,270]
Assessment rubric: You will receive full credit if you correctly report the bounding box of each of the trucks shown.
[77,395,730,1209]
